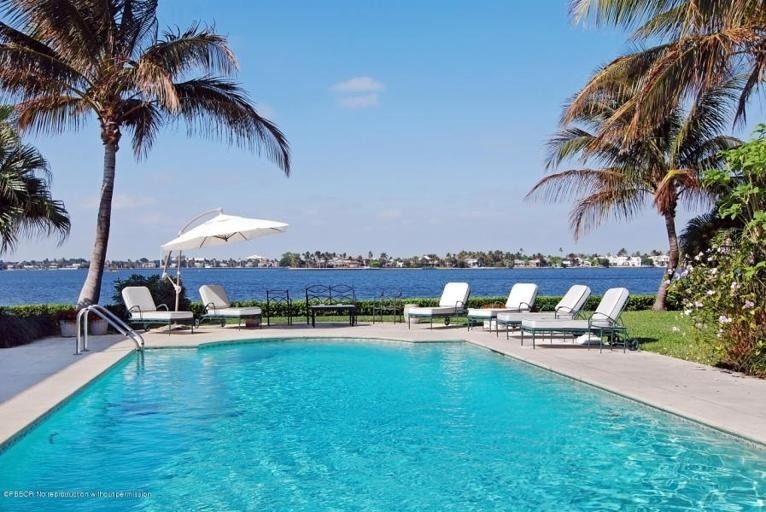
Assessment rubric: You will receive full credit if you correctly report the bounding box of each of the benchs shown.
[305,284,361,324]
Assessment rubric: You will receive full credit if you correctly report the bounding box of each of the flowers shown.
[51,306,106,322]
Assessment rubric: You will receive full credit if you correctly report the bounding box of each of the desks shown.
[308,304,356,327]
[588,321,628,355]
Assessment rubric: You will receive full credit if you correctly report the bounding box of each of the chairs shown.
[402,282,470,329]
[265,288,293,326]
[465,283,630,350]
[120,286,194,335]
[193,284,263,331]
[373,285,400,326]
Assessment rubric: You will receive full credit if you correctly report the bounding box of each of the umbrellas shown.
[162,207,290,325]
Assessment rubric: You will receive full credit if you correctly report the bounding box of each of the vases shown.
[90,320,108,335]
[59,319,77,337]
